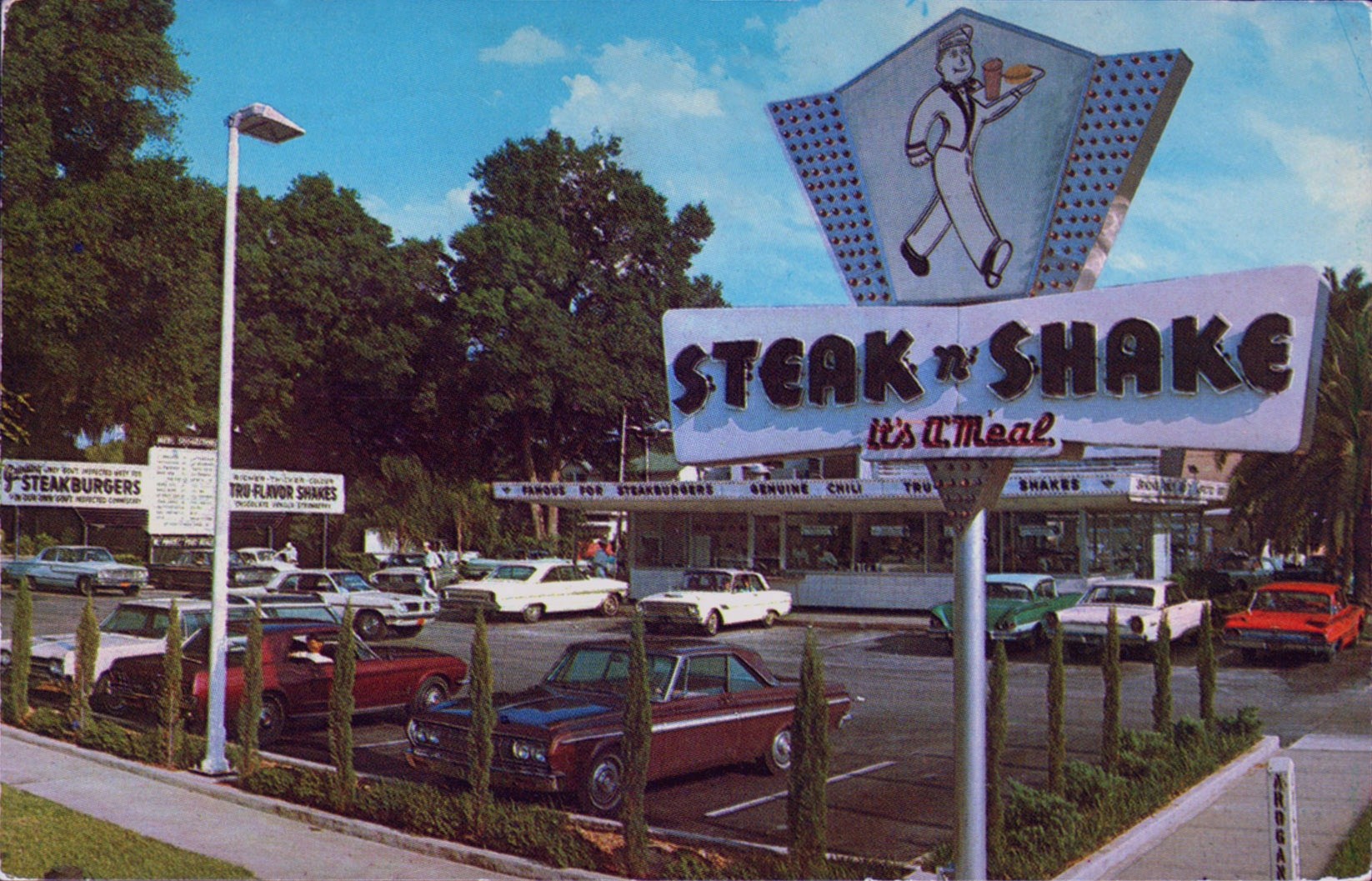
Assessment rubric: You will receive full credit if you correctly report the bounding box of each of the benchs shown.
[674,672,734,695]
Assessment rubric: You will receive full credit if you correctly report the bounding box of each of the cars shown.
[0,543,150,599]
[634,566,794,635]
[926,571,1083,655]
[436,559,630,626]
[106,618,469,751]
[1,595,263,699]
[404,634,852,821]
[1193,551,1343,596]
[1038,578,1213,663]
[1220,581,1366,663]
[150,543,481,645]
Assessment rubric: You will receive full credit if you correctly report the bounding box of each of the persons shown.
[422,542,441,590]
[688,574,755,594]
[596,538,629,579]
[273,542,297,566]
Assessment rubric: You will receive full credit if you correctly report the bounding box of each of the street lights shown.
[195,101,305,776]
